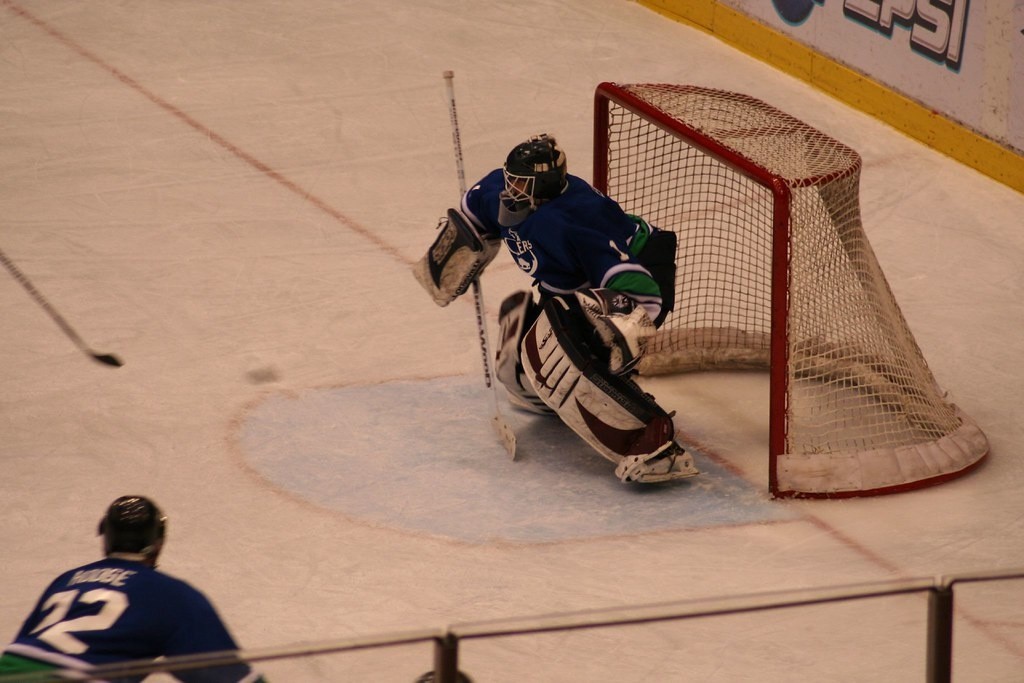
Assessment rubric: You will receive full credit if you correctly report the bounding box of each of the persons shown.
[412,133,699,491]
[0,495,269,683]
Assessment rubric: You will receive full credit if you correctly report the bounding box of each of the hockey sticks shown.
[0,246,125,370]
[440,68,522,463]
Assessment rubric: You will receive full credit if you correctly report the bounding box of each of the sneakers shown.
[624,442,698,483]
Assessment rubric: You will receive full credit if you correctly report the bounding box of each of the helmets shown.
[99,496,165,567]
[501,134,569,212]
